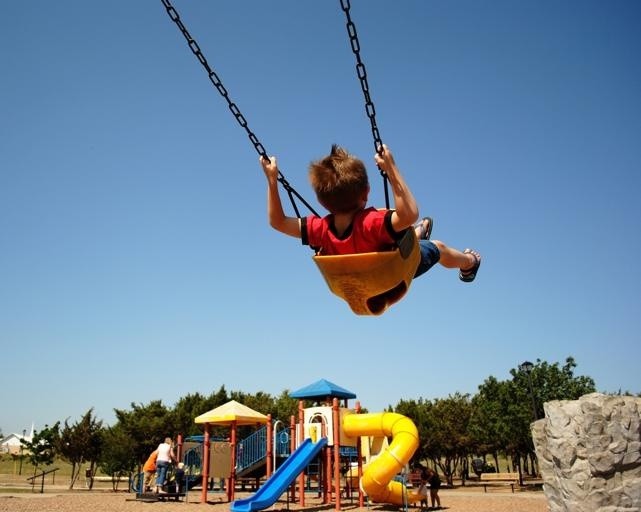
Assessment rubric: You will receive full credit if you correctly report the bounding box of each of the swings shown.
[162,0,421,316]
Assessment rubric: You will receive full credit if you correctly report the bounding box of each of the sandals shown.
[458,247,481,282]
[411,216,433,240]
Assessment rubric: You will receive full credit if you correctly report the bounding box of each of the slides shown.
[344,412,429,506]
[230,437,327,512]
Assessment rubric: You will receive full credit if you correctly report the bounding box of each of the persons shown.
[416,472,430,511]
[259,143,481,301]
[142,447,159,492]
[85,466,93,489]
[414,462,443,511]
[154,436,177,494]
